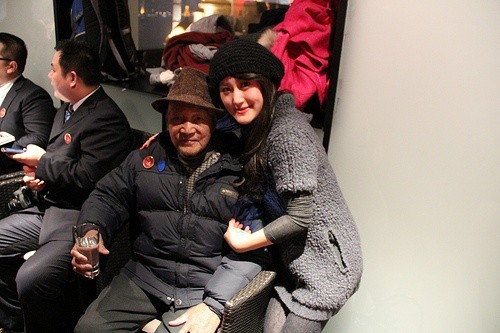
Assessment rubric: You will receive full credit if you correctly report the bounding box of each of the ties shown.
[64,105,74,124]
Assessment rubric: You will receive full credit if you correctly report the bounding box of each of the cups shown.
[72,222,100,278]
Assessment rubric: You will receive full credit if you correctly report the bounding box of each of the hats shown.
[208,39,284,99]
[151,66,226,115]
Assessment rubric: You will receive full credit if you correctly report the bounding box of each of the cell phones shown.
[1,148,24,153]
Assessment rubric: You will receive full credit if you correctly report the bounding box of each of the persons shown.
[258,0,333,106]
[0,40,134,333]
[71,66,270,333]
[208,38,363,333]
[0,31,55,219]
